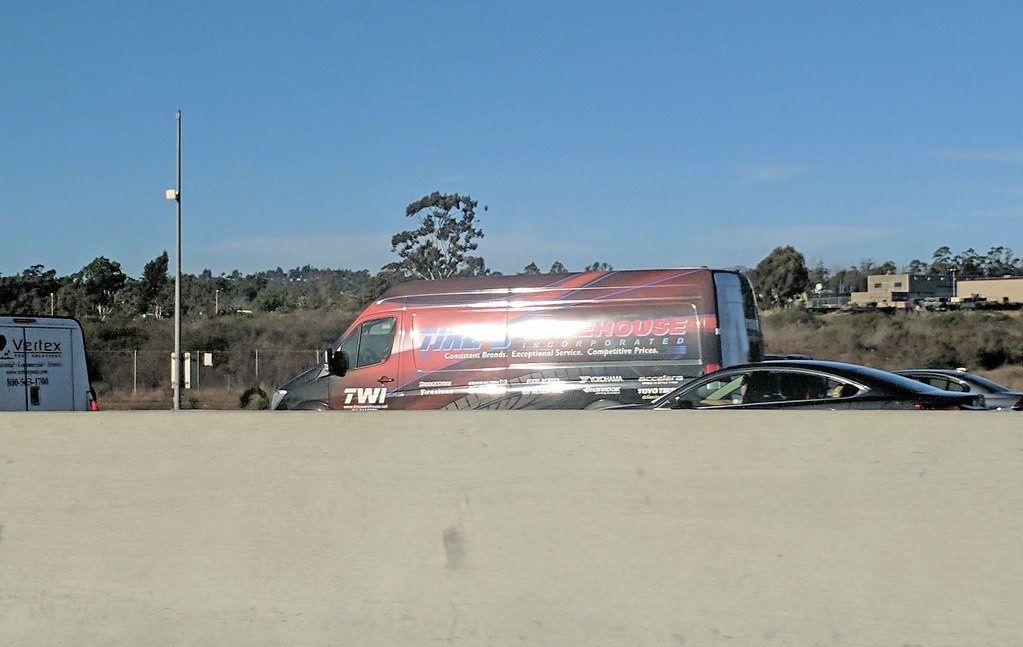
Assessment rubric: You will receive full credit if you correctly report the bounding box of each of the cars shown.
[831,368,1023,411]
[597,358,989,412]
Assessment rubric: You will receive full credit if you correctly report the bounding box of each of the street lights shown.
[164,189,182,409]
[950,268,958,297]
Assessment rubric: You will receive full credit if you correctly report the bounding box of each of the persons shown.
[700,374,750,405]
[814,282,823,296]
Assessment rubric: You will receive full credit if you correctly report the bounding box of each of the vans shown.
[267,268,762,410]
[0,316,99,410]
